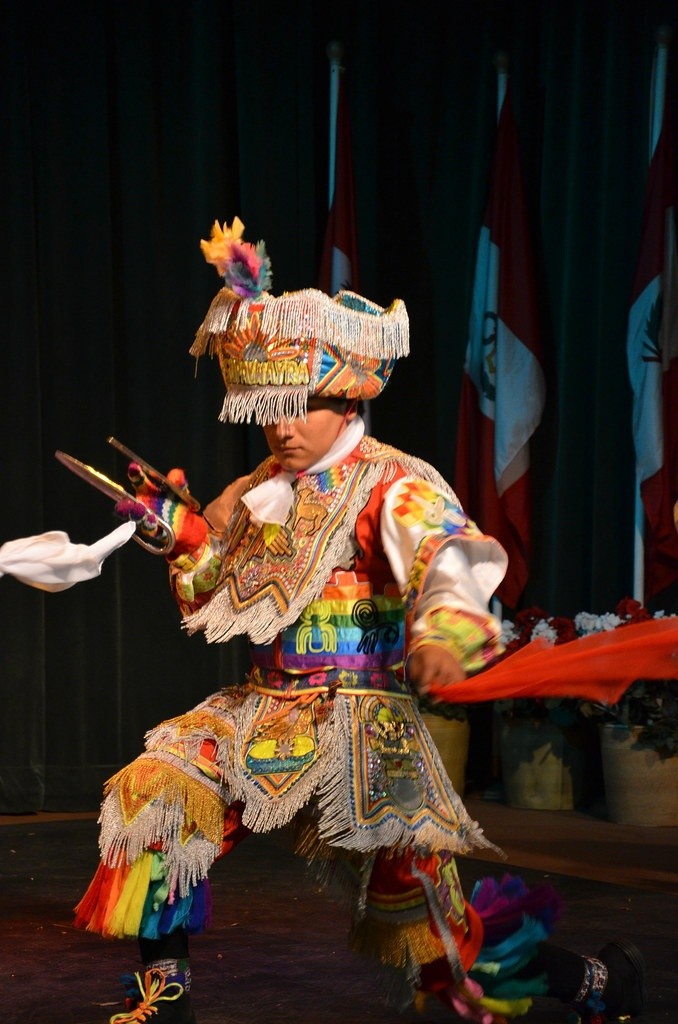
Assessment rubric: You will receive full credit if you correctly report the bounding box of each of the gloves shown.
[114,464,207,554]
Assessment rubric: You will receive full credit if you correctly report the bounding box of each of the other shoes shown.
[109,967,196,1024]
[579,936,647,1024]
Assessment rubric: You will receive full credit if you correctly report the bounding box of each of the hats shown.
[188,216,409,426]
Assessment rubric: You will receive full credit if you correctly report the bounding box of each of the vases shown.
[497,717,577,810]
[598,723,678,828]
[422,712,470,802]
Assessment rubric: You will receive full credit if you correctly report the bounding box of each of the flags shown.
[452,103,547,618]
[318,60,362,420]
[623,42,677,610]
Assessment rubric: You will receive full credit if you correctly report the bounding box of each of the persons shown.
[72,218,653,1024]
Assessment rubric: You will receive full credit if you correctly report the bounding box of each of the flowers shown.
[421,597,678,763]
